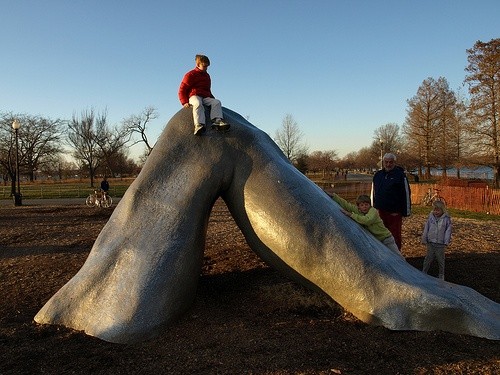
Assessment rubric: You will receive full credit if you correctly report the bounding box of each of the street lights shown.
[380,138,384,170]
[12,120,22,206]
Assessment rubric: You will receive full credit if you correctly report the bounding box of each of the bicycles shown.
[423,188,447,208]
[86,189,113,209]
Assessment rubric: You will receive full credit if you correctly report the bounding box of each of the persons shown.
[421,201,453,280]
[324,188,407,262]
[370,153,412,252]
[100,176,109,204]
[179,55,228,135]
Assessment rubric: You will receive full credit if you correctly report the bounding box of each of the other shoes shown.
[212,120,230,130]
[194,124,206,134]
